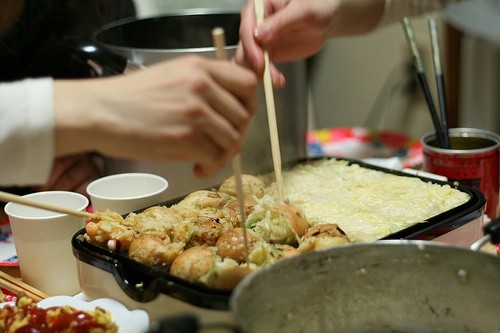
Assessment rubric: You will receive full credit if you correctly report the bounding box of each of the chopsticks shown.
[400,15,450,150]
[0,271,48,302]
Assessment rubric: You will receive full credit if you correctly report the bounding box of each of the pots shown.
[230,218,500,333]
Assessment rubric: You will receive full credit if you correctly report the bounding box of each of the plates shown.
[0,205,94,266]
[0,295,149,333]
[307,126,423,168]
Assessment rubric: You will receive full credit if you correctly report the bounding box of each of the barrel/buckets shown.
[46,8,310,203]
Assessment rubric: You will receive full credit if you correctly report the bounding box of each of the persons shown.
[0,0,103,202]
[0,54,257,187]
[235,0,468,88]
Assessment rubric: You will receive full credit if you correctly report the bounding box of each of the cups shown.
[86,173,169,216]
[4,191,89,297]
[420,127,500,221]
